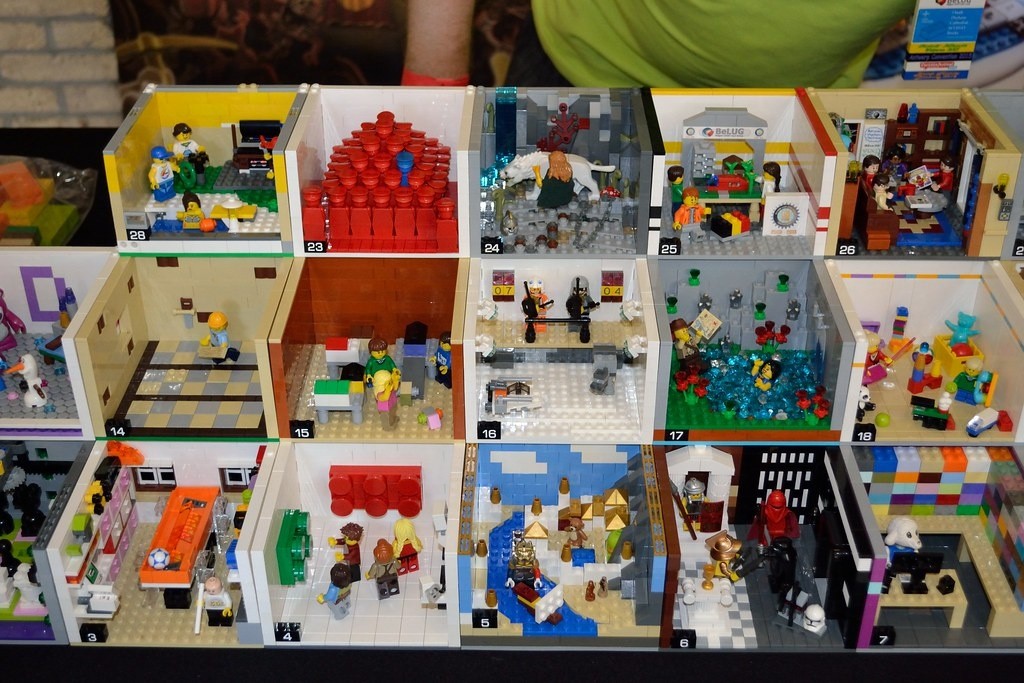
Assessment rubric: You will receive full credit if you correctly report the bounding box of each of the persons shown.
[566,276,597,332]
[148,147,182,201]
[871,171,895,211]
[678,478,800,615]
[882,147,910,181]
[669,318,702,375]
[759,162,781,229]
[389,518,423,577]
[537,150,575,208]
[953,358,983,406]
[862,154,881,197]
[365,539,401,600]
[667,165,685,222]
[364,337,396,389]
[316,563,351,620]
[751,357,783,390]
[400,0,916,110]
[672,187,712,245]
[929,153,954,194]
[195,576,234,627]
[429,331,453,389]
[328,522,364,583]
[172,123,209,164]
[864,327,892,378]
[521,280,547,333]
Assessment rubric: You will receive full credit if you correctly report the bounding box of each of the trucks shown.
[967,407,998,439]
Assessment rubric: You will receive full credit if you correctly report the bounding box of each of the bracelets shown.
[402,66,469,89]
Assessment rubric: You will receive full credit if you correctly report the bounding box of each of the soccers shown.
[148,548,170,570]
